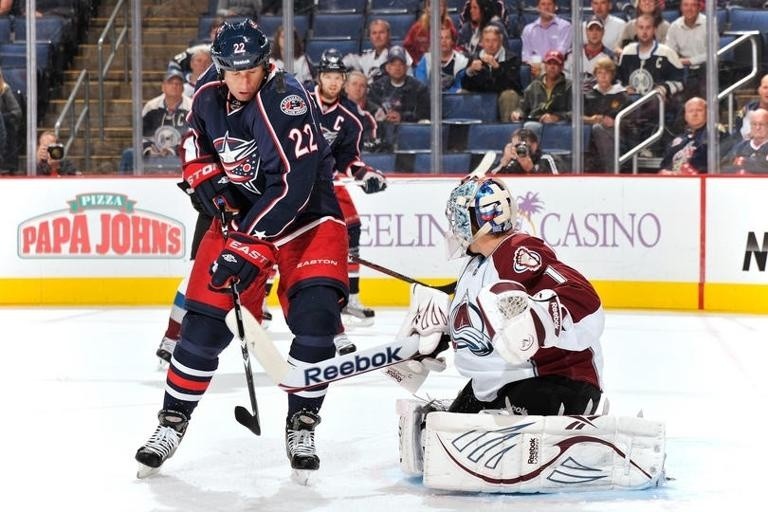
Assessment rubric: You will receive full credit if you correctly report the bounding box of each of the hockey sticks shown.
[215,198,261,436]
[224,303,449,395]
[347,254,456,298]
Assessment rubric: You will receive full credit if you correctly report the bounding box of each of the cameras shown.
[47,143,65,162]
[515,128,531,156]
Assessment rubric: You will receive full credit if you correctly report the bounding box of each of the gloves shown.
[208,231,281,303]
[347,161,388,193]
[178,155,244,226]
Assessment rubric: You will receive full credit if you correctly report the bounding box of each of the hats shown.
[541,49,565,67]
[163,69,185,83]
[585,14,605,30]
[387,45,407,65]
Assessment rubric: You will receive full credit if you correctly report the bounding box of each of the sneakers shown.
[333,331,357,355]
[157,334,176,362]
[285,410,321,469]
[341,293,375,319]
[135,411,190,466]
[260,308,272,320]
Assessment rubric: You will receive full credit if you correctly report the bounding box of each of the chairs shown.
[359,92,593,176]
[716,6,767,86]
[504,1,541,63]
[0,15,71,124]
[661,10,681,23]
[558,11,627,22]
[189,1,464,67]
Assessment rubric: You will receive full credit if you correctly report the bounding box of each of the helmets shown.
[210,19,271,81]
[445,172,518,255]
[315,48,349,75]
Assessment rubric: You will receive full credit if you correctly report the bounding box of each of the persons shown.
[37,131,76,176]
[136,18,350,468]
[254,46,386,320]
[20,1,77,25]
[386,177,666,492]
[1,0,14,16]
[156,64,356,362]
[0,72,22,122]
[0,117,9,161]
